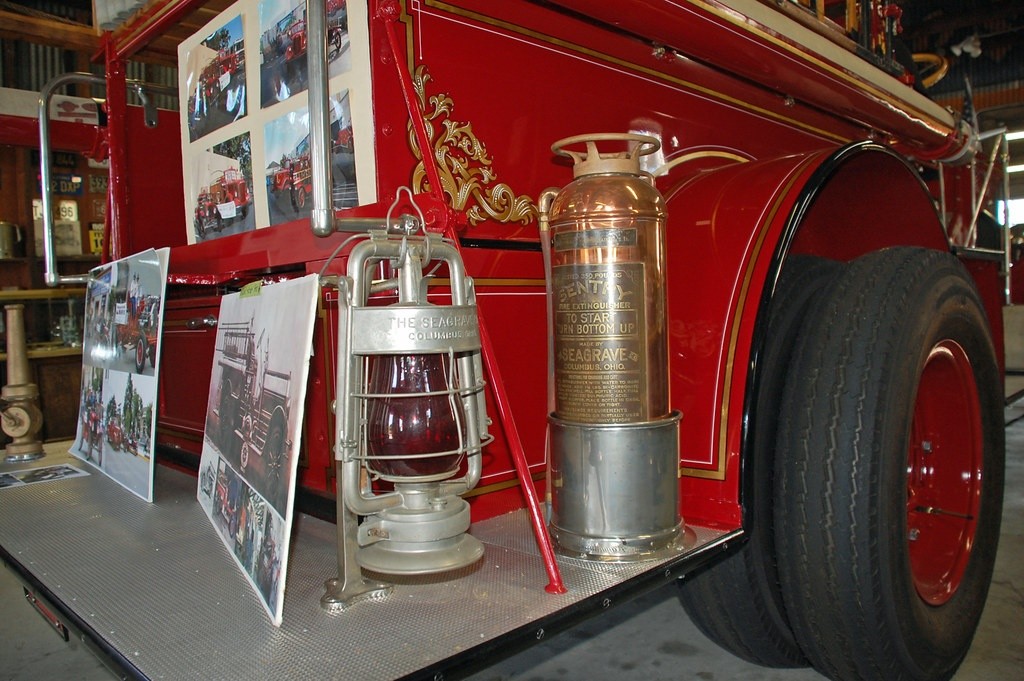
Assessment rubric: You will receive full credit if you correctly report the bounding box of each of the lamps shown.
[334,185,495,575]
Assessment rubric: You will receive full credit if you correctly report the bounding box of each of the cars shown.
[1,1,1024,680]
